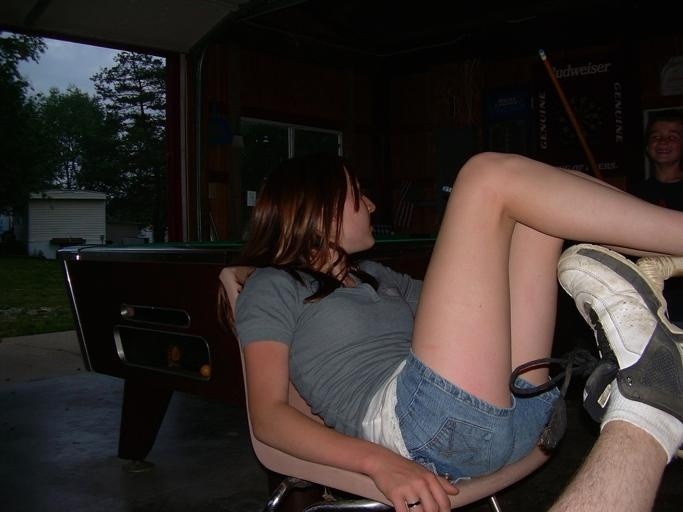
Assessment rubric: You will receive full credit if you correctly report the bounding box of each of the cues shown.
[539,50,604,182]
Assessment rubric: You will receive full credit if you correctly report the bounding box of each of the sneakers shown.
[556,243,683,425]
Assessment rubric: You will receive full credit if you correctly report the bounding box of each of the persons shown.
[546,242,683,512]
[635,109,683,212]
[232,145,683,512]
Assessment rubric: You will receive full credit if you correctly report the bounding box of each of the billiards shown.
[201,366,210,377]
[127,308,134,316]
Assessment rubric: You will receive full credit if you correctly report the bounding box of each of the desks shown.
[54,236,440,460]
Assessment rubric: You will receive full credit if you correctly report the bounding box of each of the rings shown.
[407,496,422,509]
